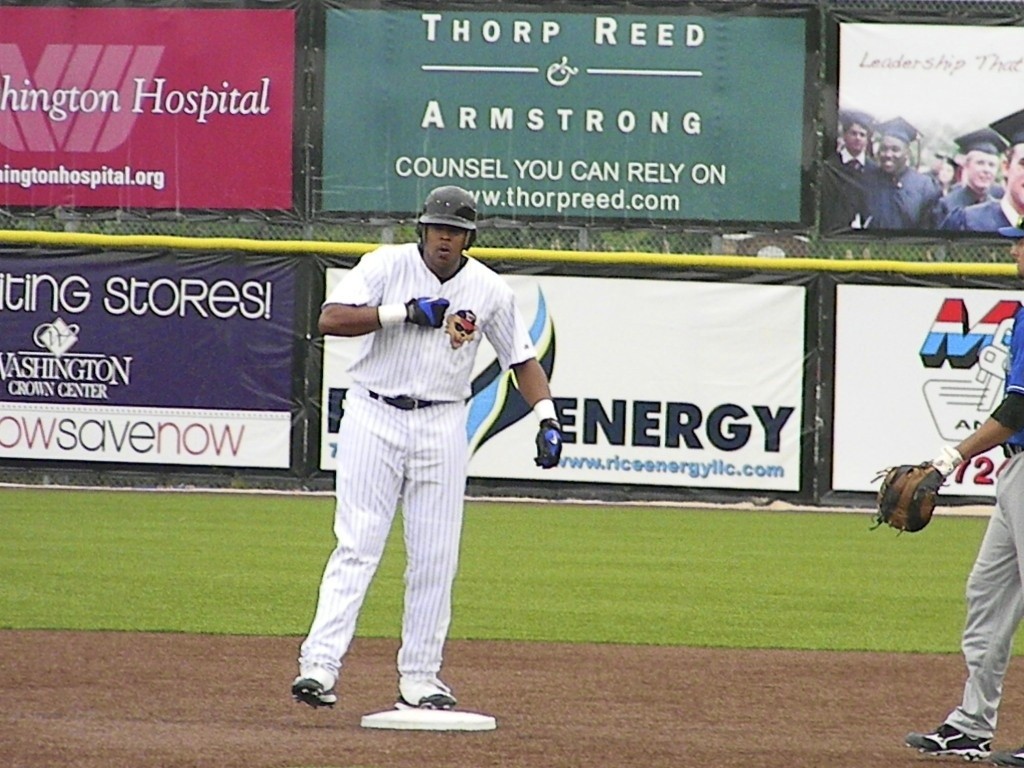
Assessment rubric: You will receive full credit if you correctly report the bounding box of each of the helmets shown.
[419,185,478,250]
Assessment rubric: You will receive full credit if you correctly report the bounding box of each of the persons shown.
[292,184,564,707]
[867,214,1024,768]
[823,105,1024,235]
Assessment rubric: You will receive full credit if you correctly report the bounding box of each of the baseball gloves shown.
[876,462,944,533]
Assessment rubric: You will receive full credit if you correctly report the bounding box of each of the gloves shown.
[405,296,450,328]
[534,418,563,469]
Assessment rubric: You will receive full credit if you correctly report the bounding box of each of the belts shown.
[368,390,456,410]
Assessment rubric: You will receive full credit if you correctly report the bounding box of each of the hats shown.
[989,109,1024,146]
[839,110,876,158]
[876,119,924,169]
[997,215,1024,238]
[954,128,1007,181]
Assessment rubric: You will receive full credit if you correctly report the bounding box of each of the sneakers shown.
[991,744,1024,768]
[291,676,335,709]
[904,724,992,762]
[394,694,457,710]
